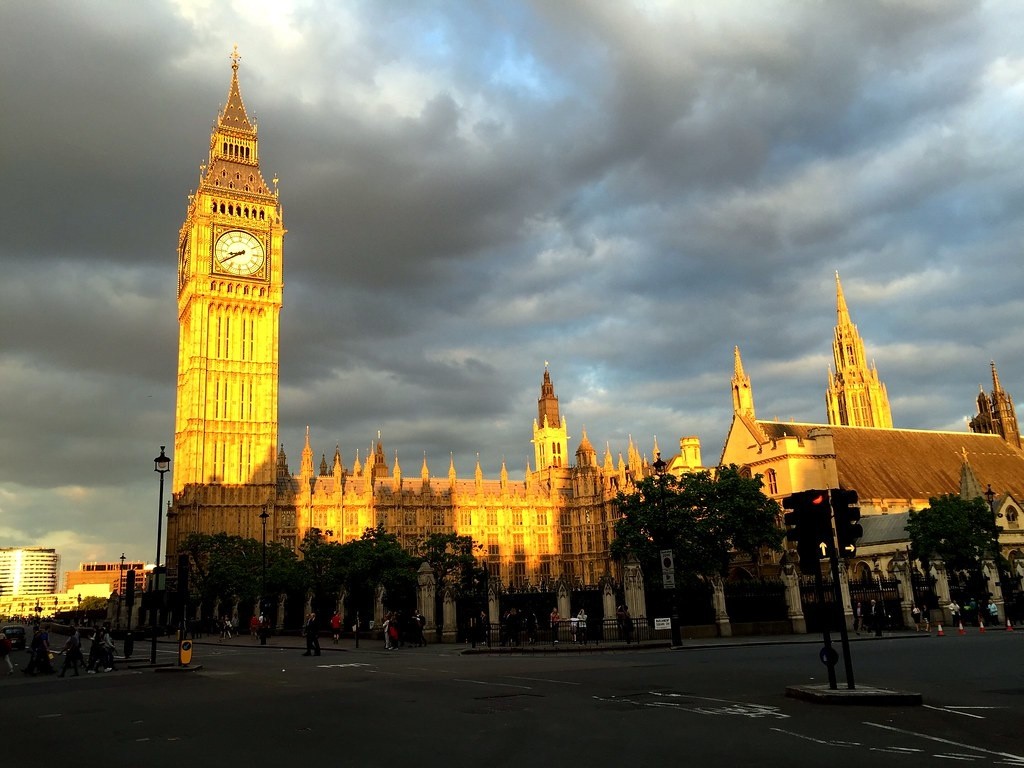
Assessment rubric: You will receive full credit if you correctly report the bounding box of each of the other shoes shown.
[88,670,96,674]
[104,668,112,672]
[8,667,16,675]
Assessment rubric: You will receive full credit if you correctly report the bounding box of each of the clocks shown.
[182,239,188,282]
[214,229,264,277]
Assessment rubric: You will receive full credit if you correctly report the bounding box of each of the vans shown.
[0,625,26,650]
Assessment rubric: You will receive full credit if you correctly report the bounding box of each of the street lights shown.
[54,597,58,614]
[985,482,1018,627]
[115,553,126,628]
[77,593,82,611]
[150,445,171,664]
[652,452,684,648]
[257,507,269,600]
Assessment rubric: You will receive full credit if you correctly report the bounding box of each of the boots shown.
[56,668,66,678]
[71,673,79,677]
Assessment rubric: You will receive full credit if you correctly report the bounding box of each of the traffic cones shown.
[1006,618,1013,632]
[959,620,965,634]
[978,620,985,633]
[936,622,947,636]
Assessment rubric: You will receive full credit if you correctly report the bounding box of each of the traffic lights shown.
[806,489,836,559]
[781,492,807,542]
[831,488,864,557]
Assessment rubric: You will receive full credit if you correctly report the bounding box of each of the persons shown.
[550,606,561,643]
[616,605,634,644]
[1013,591,1024,627]
[185,611,240,640]
[481,606,539,646]
[331,611,341,645]
[912,603,920,632]
[985,599,1000,627]
[0,614,116,678]
[570,608,588,646]
[948,599,960,627]
[970,598,979,627]
[249,611,272,641]
[922,603,932,633]
[856,599,884,636]
[301,613,322,657]
[382,609,427,650]
